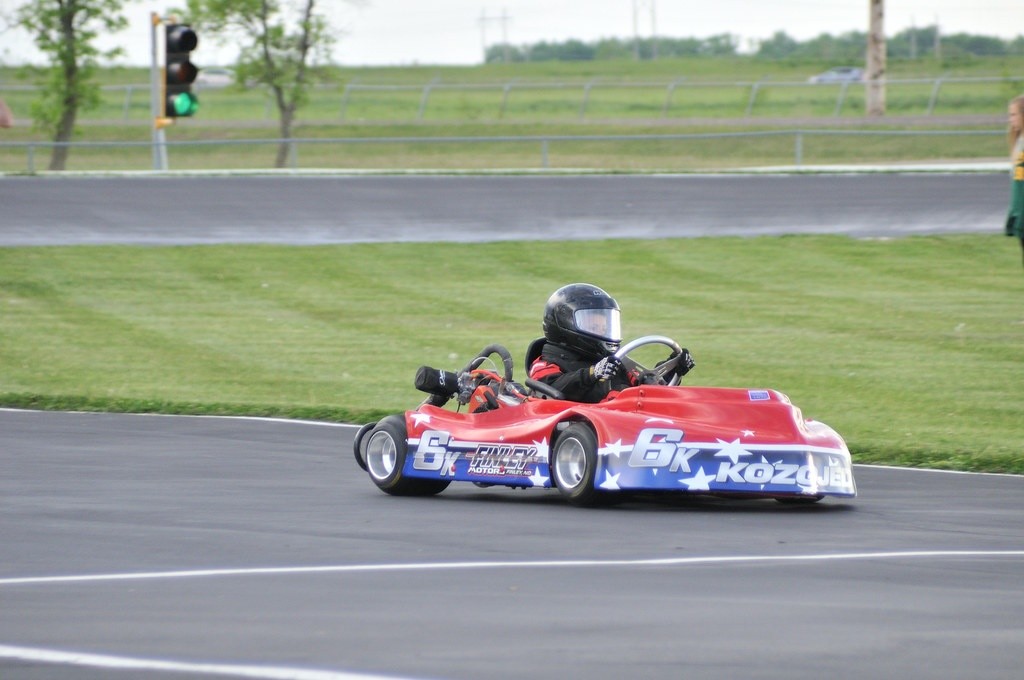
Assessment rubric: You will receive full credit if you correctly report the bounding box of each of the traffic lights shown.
[161,22,199,117]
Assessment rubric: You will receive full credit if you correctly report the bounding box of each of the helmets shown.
[543,282,623,363]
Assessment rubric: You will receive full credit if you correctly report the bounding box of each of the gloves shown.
[668,348,695,377]
[590,355,625,384]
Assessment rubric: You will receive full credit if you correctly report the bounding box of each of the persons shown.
[1005,96,1024,239]
[529,282,695,404]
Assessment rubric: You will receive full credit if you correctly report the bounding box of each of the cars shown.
[807,66,863,81]
